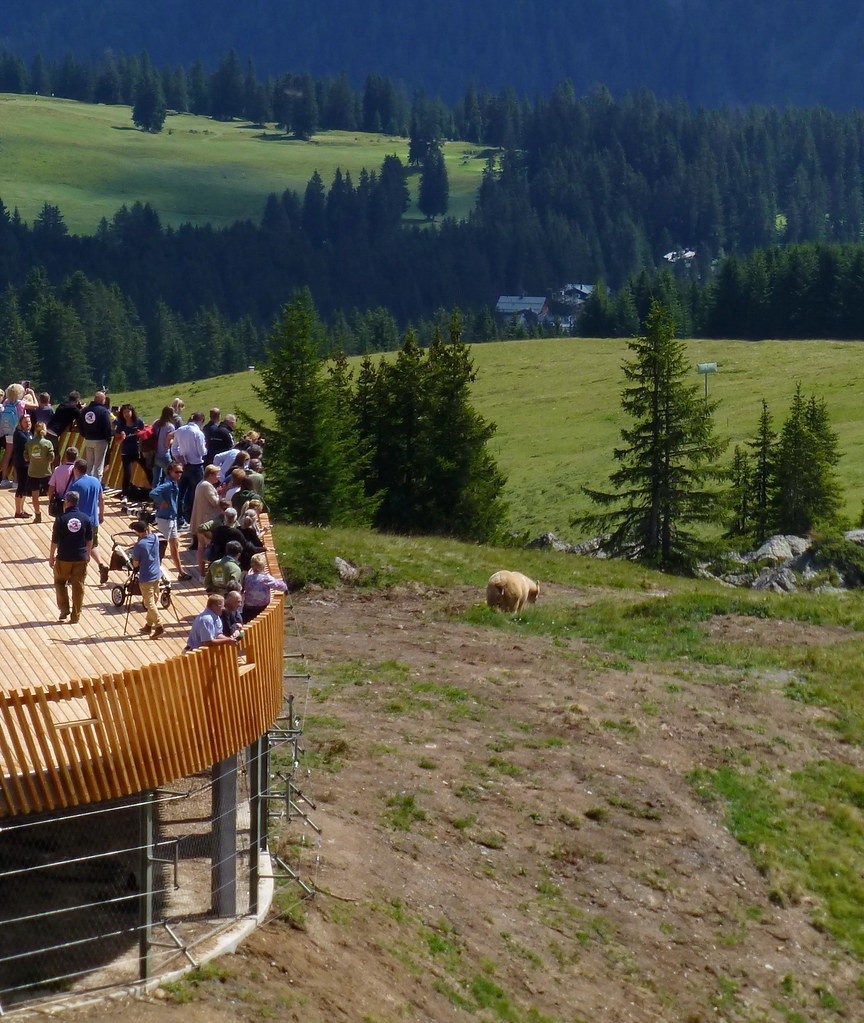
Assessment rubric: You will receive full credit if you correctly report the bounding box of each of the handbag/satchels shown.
[48,494,65,518]
[138,426,157,451]
[145,449,155,468]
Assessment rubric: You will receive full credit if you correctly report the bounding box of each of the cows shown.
[486,570,540,614]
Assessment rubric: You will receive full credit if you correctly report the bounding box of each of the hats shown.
[129,522,147,532]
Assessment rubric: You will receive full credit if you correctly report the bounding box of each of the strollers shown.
[108,530,173,609]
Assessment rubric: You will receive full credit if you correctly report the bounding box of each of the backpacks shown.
[1,400,21,435]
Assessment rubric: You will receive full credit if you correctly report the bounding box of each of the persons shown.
[50,491,94,623]
[182,595,238,653]
[128,522,166,638]
[222,581,242,612]
[220,591,242,638]
[0,380,271,575]
[204,541,242,598]
[242,554,288,623]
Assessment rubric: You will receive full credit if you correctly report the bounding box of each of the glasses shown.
[171,470,183,474]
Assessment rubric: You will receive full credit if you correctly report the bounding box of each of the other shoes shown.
[178,522,191,530]
[189,544,198,550]
[1,480,18,487]
[140,625,151,634]
[59,609,71,620]
[150,626,166,639]
[33,514,41,523]
[99,563,108,583]
[15,510,32,518]
[178,571,192,582]
